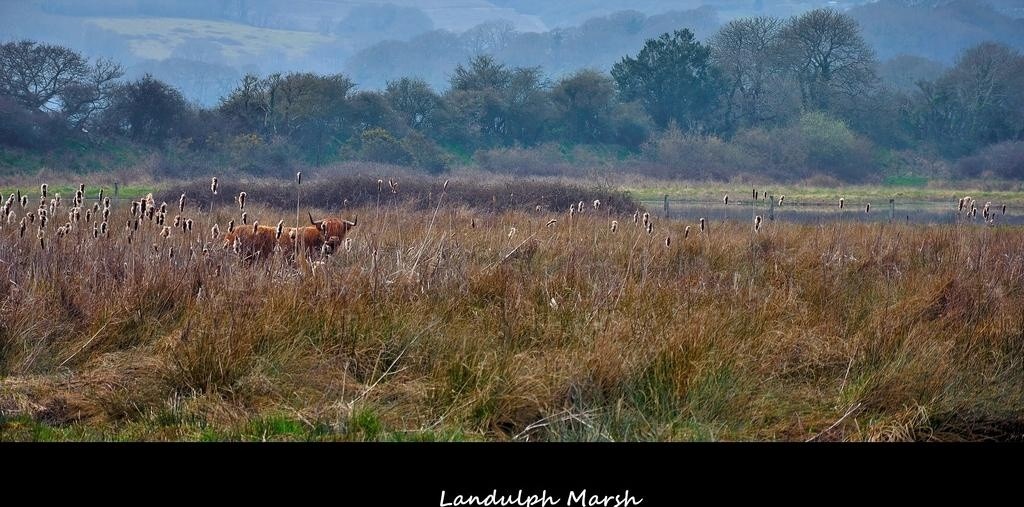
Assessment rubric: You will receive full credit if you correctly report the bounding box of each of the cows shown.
[224,211,359,268]
